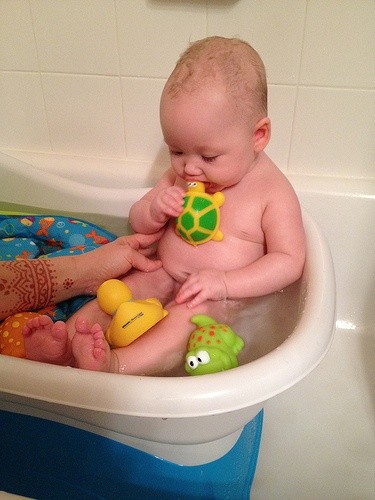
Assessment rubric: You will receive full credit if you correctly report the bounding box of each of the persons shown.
[0,223,169,319]
[21,35,307,375]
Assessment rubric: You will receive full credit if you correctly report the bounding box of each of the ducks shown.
[96,278,169,348]
